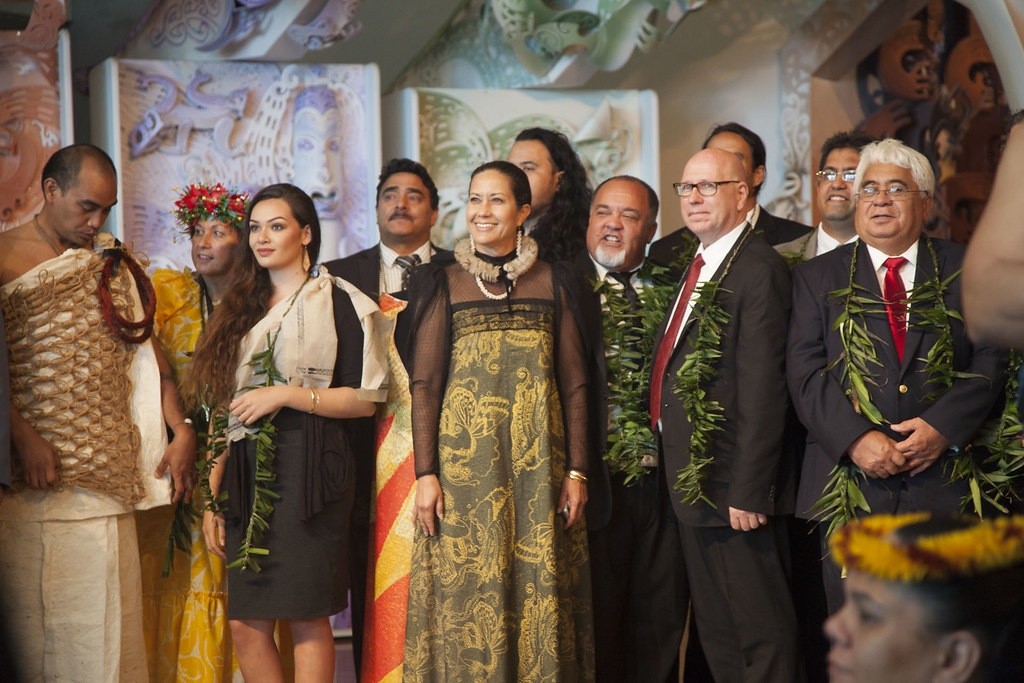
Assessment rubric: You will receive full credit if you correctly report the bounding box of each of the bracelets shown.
[172,417,192,432]
[310,386,319,415]
[567,470,589,482]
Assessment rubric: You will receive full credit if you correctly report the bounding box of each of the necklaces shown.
[470,229,521,299]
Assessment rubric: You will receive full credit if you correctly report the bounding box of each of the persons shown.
[822,518,1024,682]
[147,184,282,683]
[508,127,591,260]
[202,182,390,683]
[322,162,607,681]
[795,129,1024,589]
[567,175,691,683]
[645,122,815,683]
[0,141,197,683]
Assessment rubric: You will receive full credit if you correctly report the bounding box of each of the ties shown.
[650,253,706,432]
[882,256,908,367]
[608,268,657,456]
[395,254,422,291]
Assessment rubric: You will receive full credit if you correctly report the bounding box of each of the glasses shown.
[855,185,929,201]
[673,180,741,197]
[814,168,857,182]
